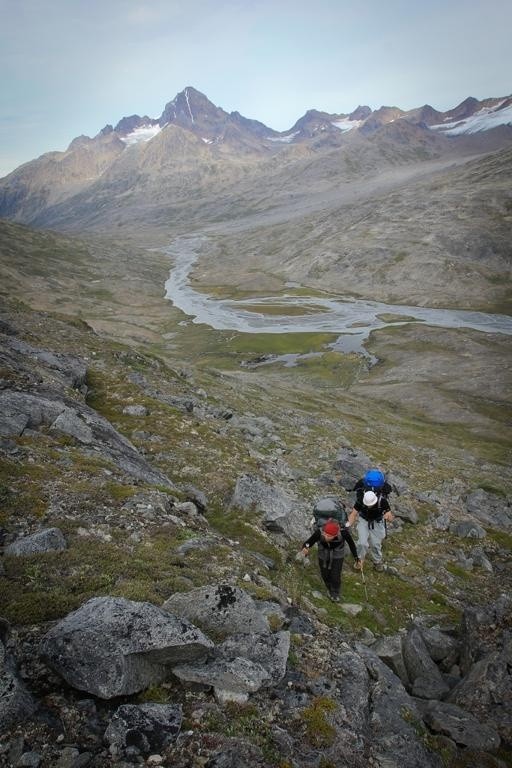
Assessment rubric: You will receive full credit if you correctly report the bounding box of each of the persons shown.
[344,489,394,573]
[299,519,365,604]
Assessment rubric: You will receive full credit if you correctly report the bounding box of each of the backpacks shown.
[314,497,348,530]
[356,469,391,508]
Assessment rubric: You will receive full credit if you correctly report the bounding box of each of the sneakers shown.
[327,590,339,602]
[354,560,364,569]
[374,563,383,571]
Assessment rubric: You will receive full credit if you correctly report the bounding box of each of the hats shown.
[323,523,339,536]
[363,491,377,506]
